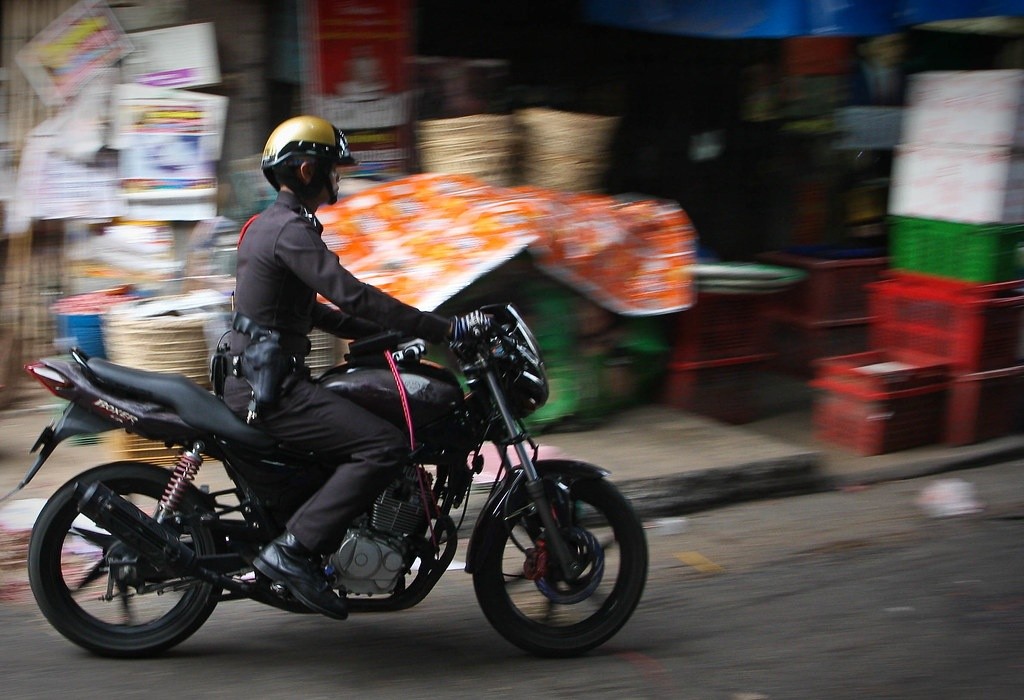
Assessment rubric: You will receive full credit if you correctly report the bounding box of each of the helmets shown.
[261,116,359,200]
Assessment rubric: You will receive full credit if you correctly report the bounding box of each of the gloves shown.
[450,310,501,350]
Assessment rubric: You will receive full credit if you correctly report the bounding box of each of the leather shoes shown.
[253,530,349,620]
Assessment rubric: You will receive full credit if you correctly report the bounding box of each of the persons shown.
[220,113,498,621]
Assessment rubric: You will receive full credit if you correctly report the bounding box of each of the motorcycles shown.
[2,302,649,660]
